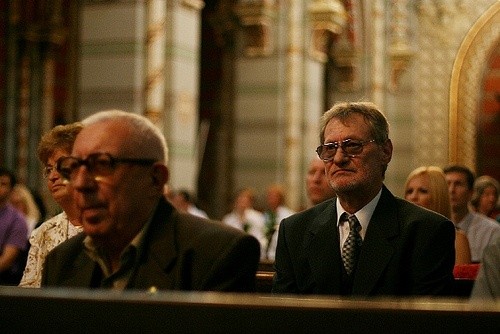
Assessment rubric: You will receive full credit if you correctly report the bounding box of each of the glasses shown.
[317,138,378,161]
[43,167,56,179]
[57,152,159,182]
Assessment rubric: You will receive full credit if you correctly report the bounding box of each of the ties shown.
[341,215,362,275]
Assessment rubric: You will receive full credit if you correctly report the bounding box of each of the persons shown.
[39,110,261,295]
[401,163,500,298]
[305,155,337,207]
[270,100,458,297]
[0,166,42,285]
[161,181,297,264]
[17,121,85,289]
